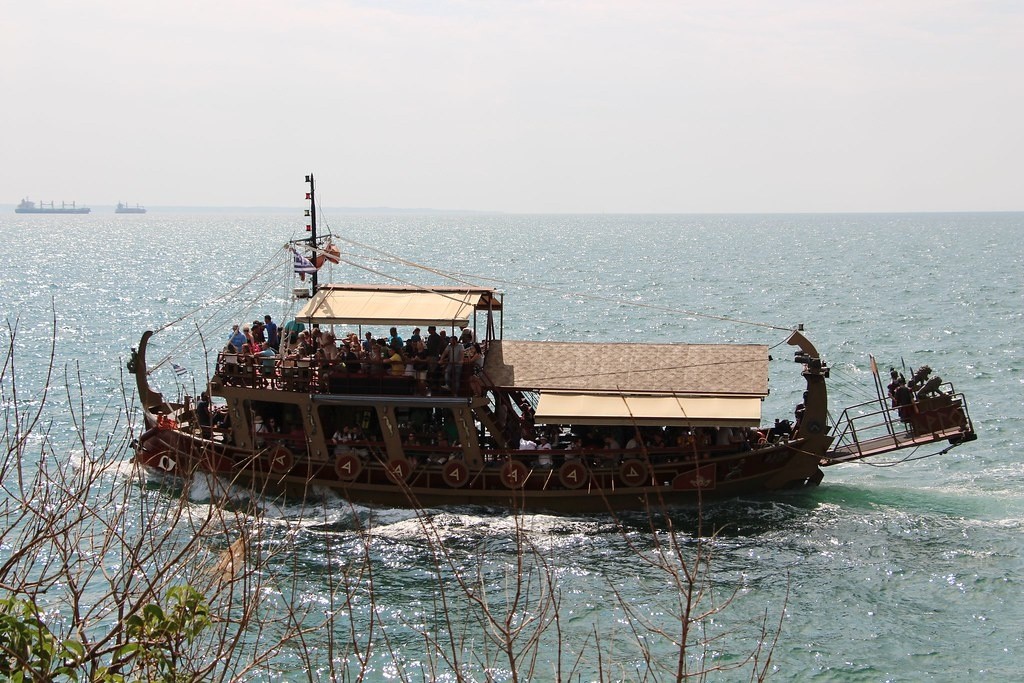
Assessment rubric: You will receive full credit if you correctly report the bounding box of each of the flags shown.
[290,249,316,274]
[171,362,187,376]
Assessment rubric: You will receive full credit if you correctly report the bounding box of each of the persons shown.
[887,371,922,437]
[197,315,751,471]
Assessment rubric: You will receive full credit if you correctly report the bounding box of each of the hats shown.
[891,371,899,377]
[899,378,905,383]
[540,436,548,439]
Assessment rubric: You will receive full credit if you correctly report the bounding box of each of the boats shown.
[126,173,979,512]
[13,196,93,215]
[113,202,147,214]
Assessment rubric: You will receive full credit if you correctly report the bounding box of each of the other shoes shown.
[663,481,669,487]
[426,390,431,397]
[441,384,450,389]
